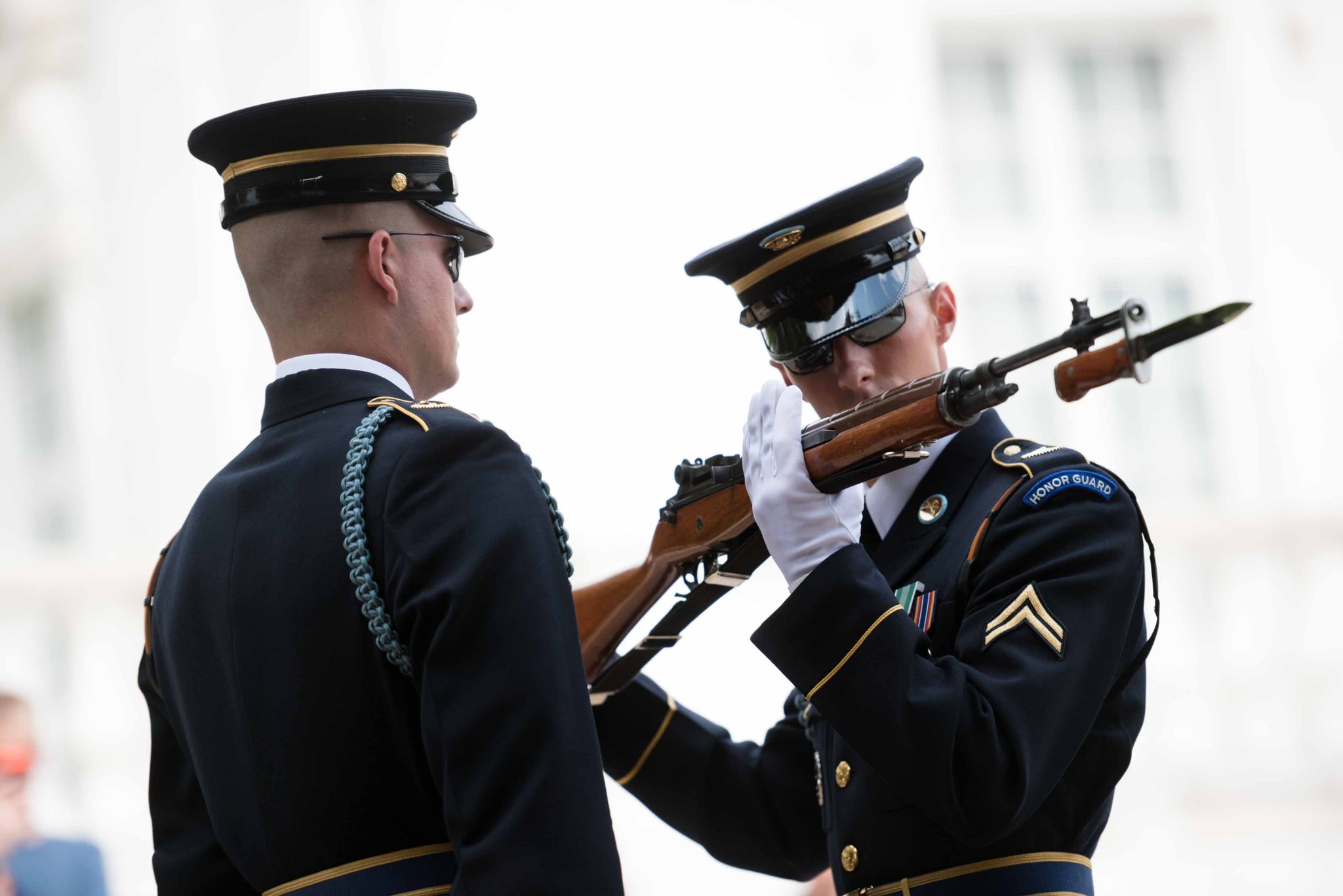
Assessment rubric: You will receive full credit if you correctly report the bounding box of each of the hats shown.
[684,157,925,363]
[188,89,493,257]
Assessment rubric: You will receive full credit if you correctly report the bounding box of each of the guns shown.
[573,293,1253,698]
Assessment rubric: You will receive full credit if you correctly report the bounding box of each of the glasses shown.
[781,284,930,375]
[322,231,463,283]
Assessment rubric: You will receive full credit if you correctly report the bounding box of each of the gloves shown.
[741,381,865,592]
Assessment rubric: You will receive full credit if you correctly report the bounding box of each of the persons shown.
[589,158,1161,896]
[138,88,626,896]
